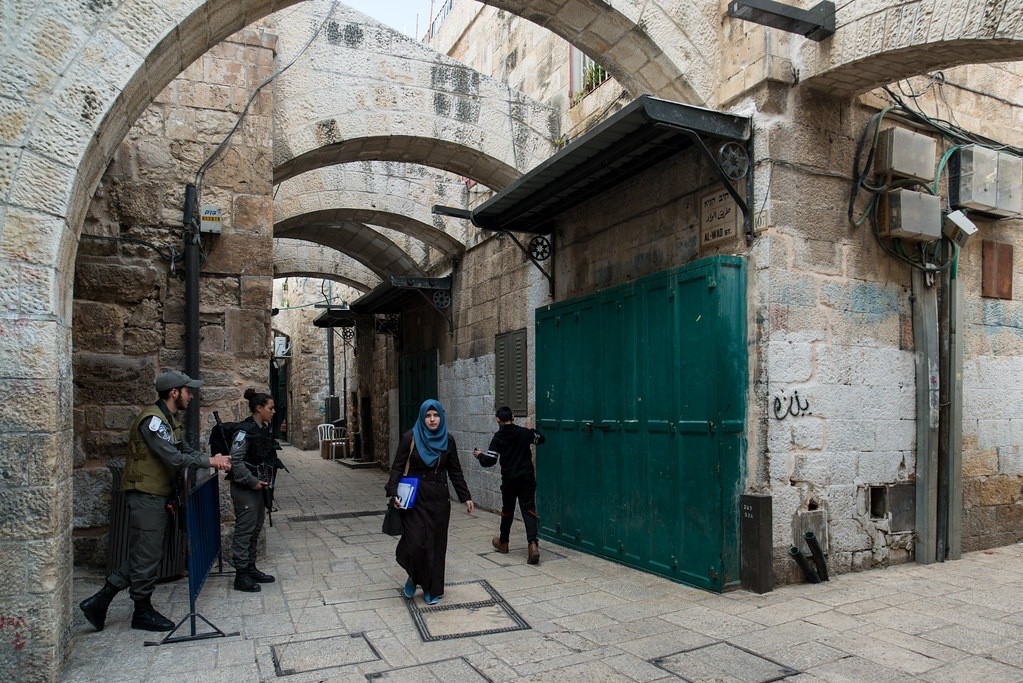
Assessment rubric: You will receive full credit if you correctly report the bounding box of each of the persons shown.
[381,398,474,605]
[472,406,547,565]
[78,370,233,633]
[226,388,279,591]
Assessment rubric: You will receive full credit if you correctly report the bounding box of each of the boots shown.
[527,541,540,564]
[131,598,175,632]
[492,537,508,553]
[79,582,120,630]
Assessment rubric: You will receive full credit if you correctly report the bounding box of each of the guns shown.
[244,462,278,528]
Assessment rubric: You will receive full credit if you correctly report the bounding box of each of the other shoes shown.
[424,591,438,604]
[403,577,416,597]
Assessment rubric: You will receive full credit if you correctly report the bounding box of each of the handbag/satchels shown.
[382,496,405,536]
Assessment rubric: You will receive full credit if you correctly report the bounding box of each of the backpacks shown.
[208,421,237,457]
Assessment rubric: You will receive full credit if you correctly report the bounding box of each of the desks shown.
[322,438,349,459]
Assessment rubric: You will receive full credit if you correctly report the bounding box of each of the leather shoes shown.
[234,572,261,592]
[248,568,275,583]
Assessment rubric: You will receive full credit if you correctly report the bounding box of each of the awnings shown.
[469,92,759,295]
[348,274,458,337]
[312,307,374,356]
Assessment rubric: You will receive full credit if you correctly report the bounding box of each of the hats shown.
[155,370,204,391]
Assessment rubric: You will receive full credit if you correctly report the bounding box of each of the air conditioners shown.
[273,336,286,357]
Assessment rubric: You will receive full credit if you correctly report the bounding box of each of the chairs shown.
[317,424,334,457]
[329,427,347,461]
